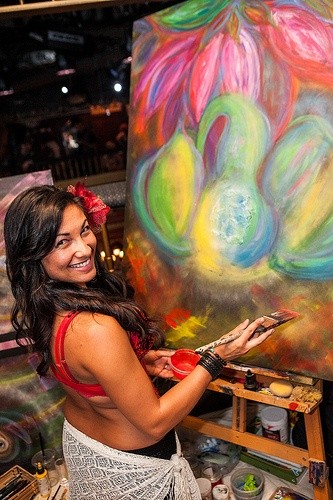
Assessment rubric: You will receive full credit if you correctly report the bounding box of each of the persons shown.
[3,185,275,499]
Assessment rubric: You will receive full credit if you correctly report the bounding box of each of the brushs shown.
[195,309,299,355]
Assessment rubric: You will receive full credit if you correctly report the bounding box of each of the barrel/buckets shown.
[230,468,265,500]
[260,406,289,444]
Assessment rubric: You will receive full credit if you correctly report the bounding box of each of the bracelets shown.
[196,349,227,382]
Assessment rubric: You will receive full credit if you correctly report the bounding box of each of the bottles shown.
[36,462,51,498]
[196,478,212,500]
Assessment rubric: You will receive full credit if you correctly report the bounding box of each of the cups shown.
[54,458,69,487]
[170,349,201,381]
[201,463,223,494]
[212,484,229,500]
[31,449,58,487]
